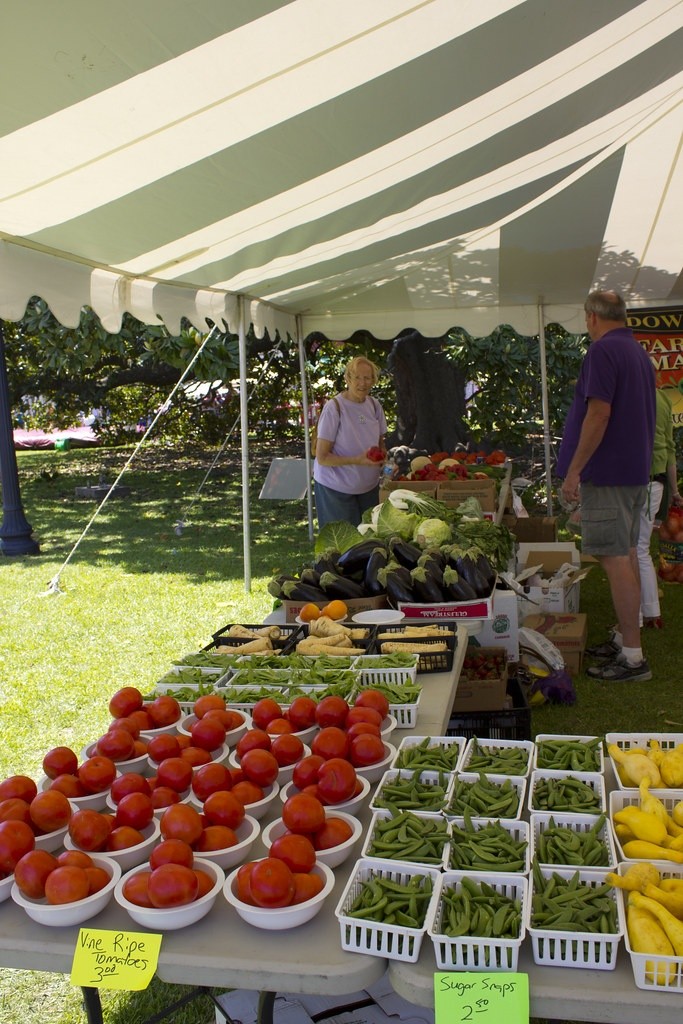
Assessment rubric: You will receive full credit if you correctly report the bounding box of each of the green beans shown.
[144,650,422,728]
[343,733,619,963]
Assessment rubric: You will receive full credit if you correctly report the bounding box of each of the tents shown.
[0,0,683,594]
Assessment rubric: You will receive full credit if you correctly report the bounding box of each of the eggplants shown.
[265,536,494,603]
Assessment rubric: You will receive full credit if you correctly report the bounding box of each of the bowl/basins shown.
[0,871,18,903]
[114,857,224,930]
[222,857,336,929]
[10,856,122,925]
[34,802,83,853]
[69,768,123,811]
[351,610,405,626]
[294,614,349,624]
[80,708,397,856]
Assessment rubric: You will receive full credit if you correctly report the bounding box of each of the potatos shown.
[656,512,683,583]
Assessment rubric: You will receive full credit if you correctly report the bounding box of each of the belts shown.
[649,474,665,482]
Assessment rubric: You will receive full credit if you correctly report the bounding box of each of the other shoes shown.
[642,616,664,630]
[606,623,623,635]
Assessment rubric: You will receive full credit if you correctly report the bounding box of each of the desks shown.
[0,621,468,1023]
[391,753,683,1023]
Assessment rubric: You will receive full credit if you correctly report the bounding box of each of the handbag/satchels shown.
[658,505,683,583]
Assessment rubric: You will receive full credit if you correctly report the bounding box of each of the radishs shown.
[212,616,453,670]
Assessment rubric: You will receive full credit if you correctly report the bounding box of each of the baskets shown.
[140,622,458,729]
[333,732,683,992]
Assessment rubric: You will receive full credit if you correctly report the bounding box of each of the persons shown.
[607,387,683,633]
[554,291,656,685]
[313,356,389,533]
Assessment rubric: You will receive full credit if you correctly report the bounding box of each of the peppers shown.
[399,463,474,481]
[368,446,385,461]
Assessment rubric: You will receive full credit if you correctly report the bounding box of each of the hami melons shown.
[411,457,432,472]
[438,458,460,470]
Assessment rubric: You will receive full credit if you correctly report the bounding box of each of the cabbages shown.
[314,489,515,576]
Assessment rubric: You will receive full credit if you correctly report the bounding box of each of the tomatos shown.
[431,450,505,464]
[0,687,390,909]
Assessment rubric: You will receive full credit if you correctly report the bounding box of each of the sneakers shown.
[585,639,621,660]
[585,655,652,683]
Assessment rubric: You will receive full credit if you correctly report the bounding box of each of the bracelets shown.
[672,492,679,496]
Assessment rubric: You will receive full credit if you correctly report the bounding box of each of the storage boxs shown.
[523,552,593,614]
[454,647,511,715]
[279,477,499,623]
[215,969,437,1024]
[519,612,592,673]
[470,680,533,742]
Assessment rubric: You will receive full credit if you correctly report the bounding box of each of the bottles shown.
[378,457,394,489]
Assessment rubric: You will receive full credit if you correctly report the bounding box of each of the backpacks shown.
[310,396,376,457]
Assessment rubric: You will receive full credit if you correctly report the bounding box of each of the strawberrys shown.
[460,652,505,681]
